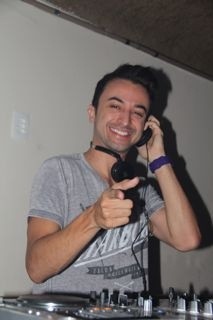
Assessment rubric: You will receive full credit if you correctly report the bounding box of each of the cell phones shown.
[136,127,152,147]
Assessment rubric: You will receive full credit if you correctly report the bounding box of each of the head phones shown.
[90,141,147,182]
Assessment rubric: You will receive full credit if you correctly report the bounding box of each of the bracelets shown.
[148,156,172,174]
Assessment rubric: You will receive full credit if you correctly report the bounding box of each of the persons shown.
[26,64,203,298]
